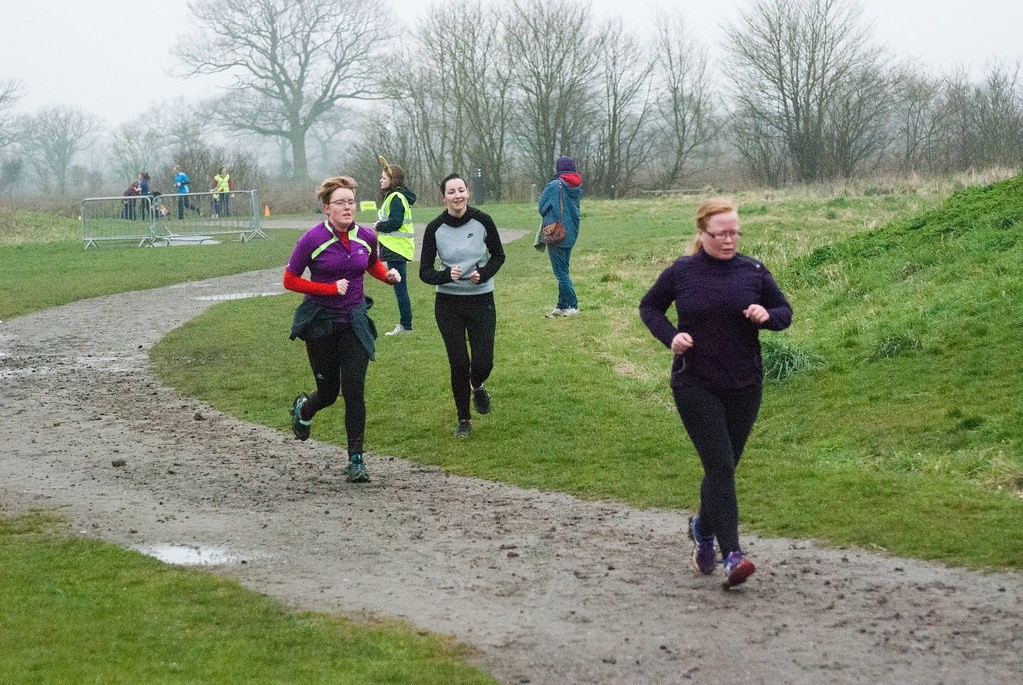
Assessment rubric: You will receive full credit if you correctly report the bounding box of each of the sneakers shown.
[473,386,492,414]
[456,419,472,438]
[293,394,312,440]
[545,308,565,316]
[347,453,370,482]
[385,324,412,335]
[564,307,580,316]
[688,516,717,573]
[721,551,755,586]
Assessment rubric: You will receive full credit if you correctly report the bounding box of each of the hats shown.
[554,157,576,175]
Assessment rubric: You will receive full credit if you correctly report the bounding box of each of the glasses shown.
[702,226,744,241]
[328,199,358,205]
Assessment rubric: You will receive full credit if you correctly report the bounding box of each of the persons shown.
[211,167,233,217]
[122,172,150,221]
[173,165,203,219]
[371,165,417,336]
[284,175,403,481]
[419,173,505,436]
[640,199,793,590]
[538,156,583,316]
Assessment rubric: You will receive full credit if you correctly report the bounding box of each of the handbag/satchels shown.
[542,223,565,244]
